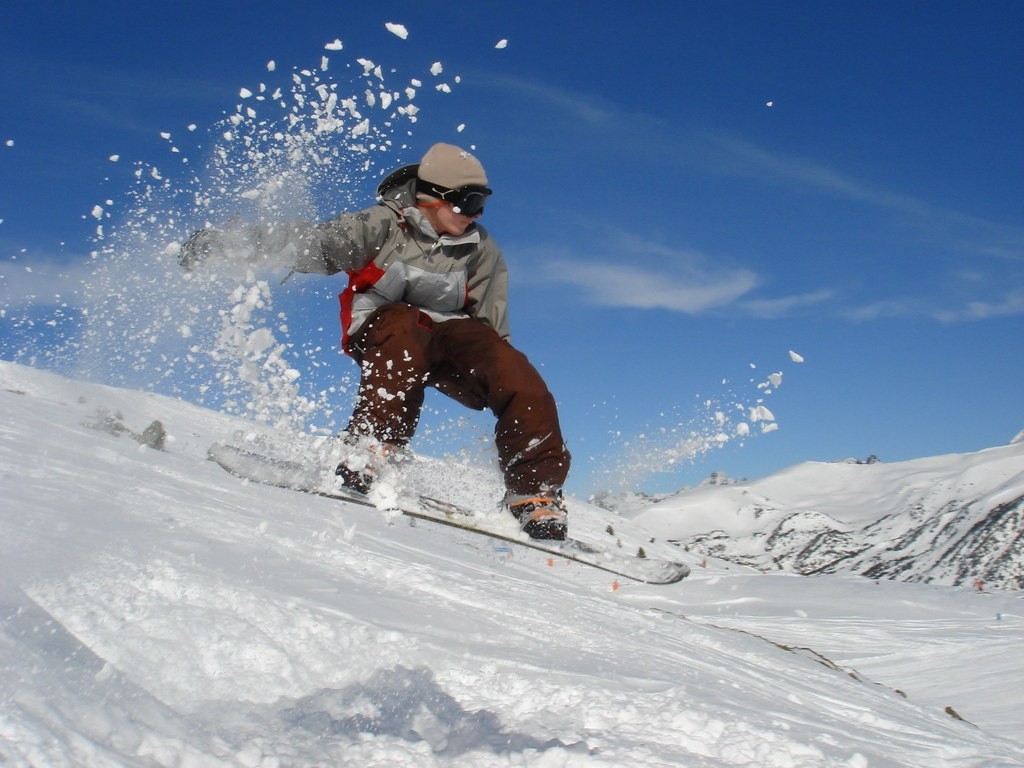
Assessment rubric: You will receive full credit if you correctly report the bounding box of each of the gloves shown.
[178,230,233,272]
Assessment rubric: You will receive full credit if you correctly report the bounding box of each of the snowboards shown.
[205,435,692,585]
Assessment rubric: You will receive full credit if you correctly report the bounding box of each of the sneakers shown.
[335,439,389,494]
[504,493,567,540]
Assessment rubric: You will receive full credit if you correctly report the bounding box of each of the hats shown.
[416,142,488,205]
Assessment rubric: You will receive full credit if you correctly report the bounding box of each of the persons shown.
[177,141,572,540]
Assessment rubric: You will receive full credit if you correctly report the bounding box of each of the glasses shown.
[453,183,493,218]
